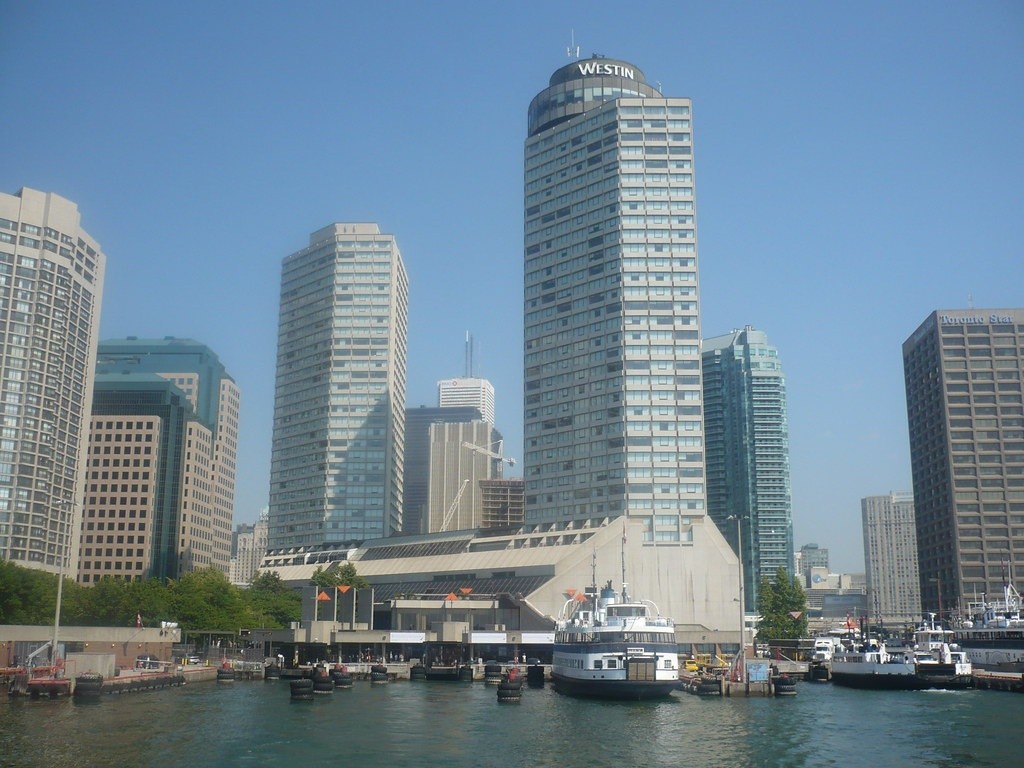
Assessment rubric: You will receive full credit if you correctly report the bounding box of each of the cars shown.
[681,660,698,671]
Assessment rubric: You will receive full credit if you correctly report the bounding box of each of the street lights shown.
[727,513,750,683]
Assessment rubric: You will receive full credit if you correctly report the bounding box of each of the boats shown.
[831,549,1024,691]
[550,520,678,699]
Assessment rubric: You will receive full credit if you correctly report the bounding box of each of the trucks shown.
[813,637,840,664]
